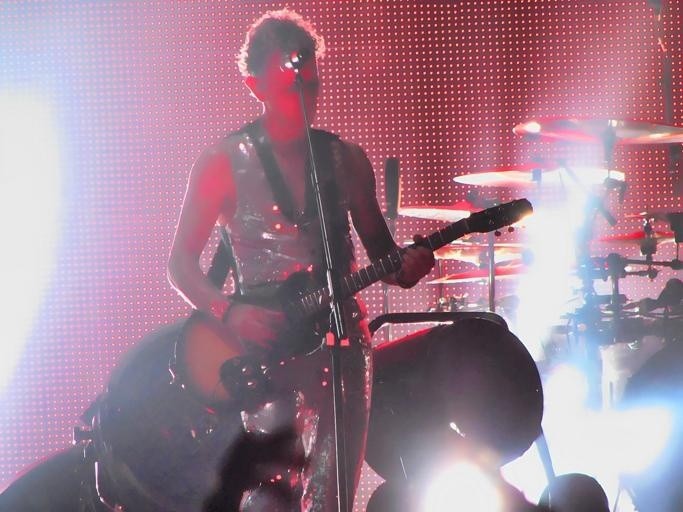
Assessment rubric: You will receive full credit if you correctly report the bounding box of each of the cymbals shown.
[511,118,683,144]
[453,163,625,187]
[398,204,498,223]
[601,235,680,246]
[427,267,528,287]
[431,242,527,266]
[626,213,673,221]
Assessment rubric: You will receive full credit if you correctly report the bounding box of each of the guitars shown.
[169,198,535,407]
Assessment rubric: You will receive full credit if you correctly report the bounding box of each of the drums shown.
[73,318,245,512]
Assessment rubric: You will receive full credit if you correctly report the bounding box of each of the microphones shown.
[278,50,308,73]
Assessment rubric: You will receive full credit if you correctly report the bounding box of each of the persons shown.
[168,7,433,511]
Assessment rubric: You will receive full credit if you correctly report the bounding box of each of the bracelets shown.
[396,268,421,290]
[221,301,240,328]
